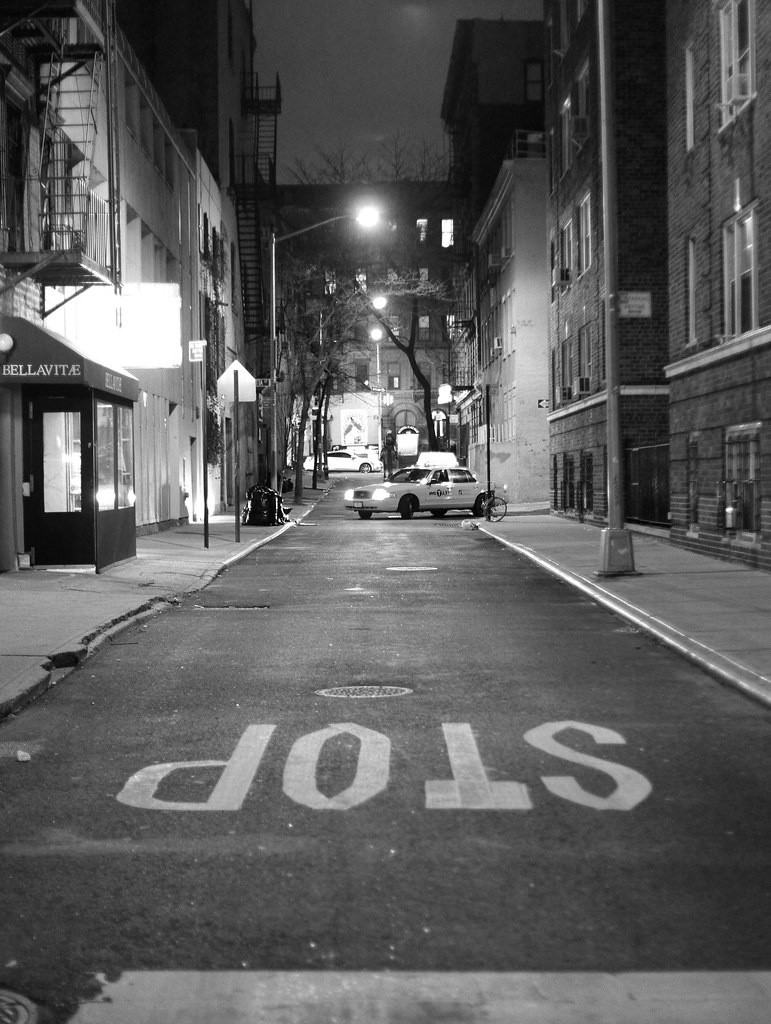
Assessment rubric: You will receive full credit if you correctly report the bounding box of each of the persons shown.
[432,472,442,483]
[380,430,398,461]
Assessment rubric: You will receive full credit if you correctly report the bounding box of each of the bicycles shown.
[482,479,507,523]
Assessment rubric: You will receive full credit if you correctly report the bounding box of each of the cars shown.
[303,450,384,473]
[343,463,489,520]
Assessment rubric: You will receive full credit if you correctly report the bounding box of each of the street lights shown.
[270,206,380,487]
